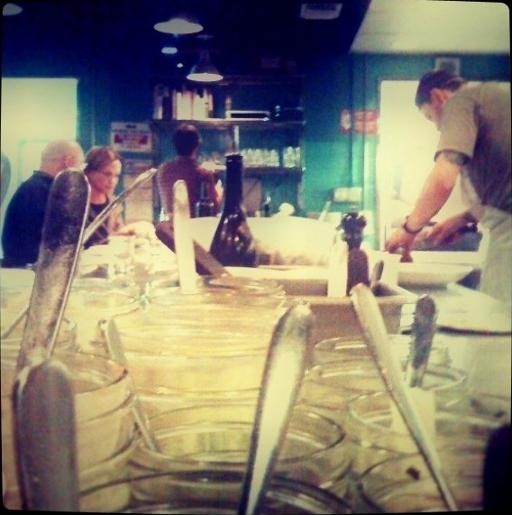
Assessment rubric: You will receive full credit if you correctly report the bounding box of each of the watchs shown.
[401,217,422,236]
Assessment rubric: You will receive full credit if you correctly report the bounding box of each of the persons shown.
[156,123,219,223]
[384,71,512,312]
[83,145,156,249]
[1,141,87,269]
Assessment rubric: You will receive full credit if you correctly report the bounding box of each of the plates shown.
[387,263,474,286]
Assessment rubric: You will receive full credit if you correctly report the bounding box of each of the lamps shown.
[186,39,222,82]
[155,18,203,35]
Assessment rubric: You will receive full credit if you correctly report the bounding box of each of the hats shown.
[173,123,200,147]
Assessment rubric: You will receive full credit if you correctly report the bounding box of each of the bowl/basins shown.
[0,343,136,512]
[248,276,420,340]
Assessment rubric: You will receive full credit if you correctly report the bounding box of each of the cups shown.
[311,335,453,368]
[110,311,302,399]
[359,452,485,514]
[345,390,511,449]
[77,470,356,514]
[143,275,289,313]
[303,356,470,412]
[106,232,135,279]
[134,401,352,501]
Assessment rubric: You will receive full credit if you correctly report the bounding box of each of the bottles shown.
[209,154,258,267]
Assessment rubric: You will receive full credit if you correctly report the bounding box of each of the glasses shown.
[97,168,122,178]
